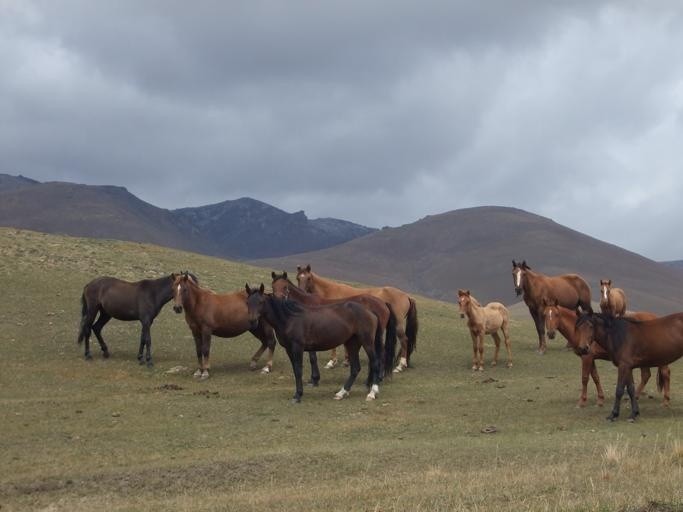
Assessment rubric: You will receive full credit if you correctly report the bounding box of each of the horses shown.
[296,264,418,373]
[170,270,277,380]
[541,297,671,409]
[457,288,513,374]
[512,260,593,354]
[272,270,398,388]
[77,271,199,368]
[574,304,683,424]
[600,279,626,317]
[245,281,382,404]
